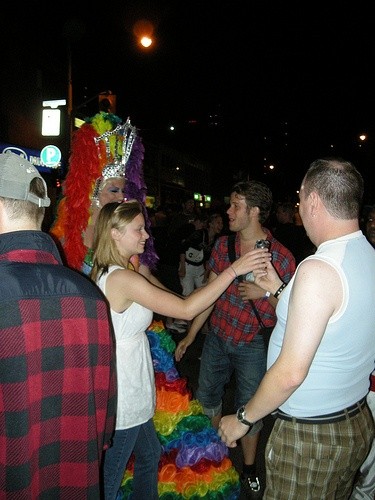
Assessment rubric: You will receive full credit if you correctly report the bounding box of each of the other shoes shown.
[243,474,262,499]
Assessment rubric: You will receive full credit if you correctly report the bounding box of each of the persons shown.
[216,160,375,500]
[0,151,118,500]
[53,112,375,500]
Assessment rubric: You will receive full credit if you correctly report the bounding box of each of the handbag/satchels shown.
[260,327,274,350]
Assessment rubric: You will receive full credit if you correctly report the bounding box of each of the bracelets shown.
[223,266,238,279]
[273,282,287,298]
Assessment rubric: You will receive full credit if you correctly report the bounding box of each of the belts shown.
[276,400,370,425]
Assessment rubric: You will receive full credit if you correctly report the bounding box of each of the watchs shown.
[262,291,271,300]
[237,407,254,426]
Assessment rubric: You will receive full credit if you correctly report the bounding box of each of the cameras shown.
[254,239,272,265]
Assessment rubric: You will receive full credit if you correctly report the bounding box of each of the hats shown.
[0,153,51,207]
